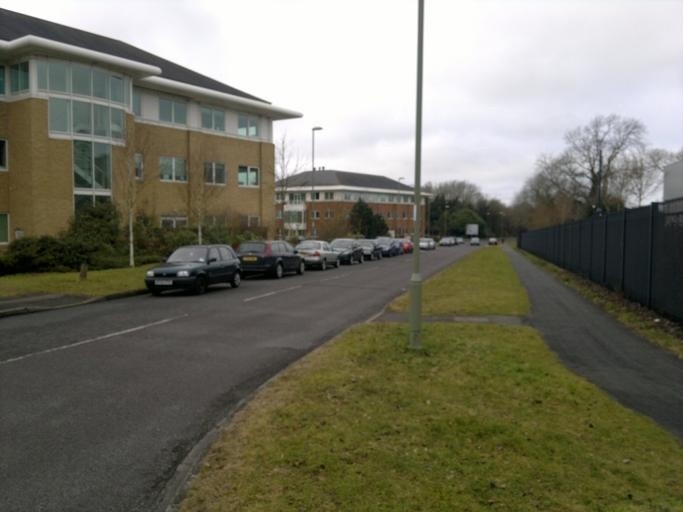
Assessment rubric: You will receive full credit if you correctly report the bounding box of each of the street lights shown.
[312,126,323,240]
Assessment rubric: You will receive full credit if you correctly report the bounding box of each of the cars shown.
[236,239,305,280]
[330,235,415,266]
[143,243,241,297]
[295,239,341,270]
[419,236,498,250]
